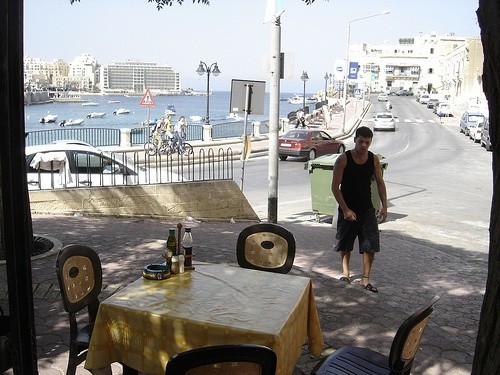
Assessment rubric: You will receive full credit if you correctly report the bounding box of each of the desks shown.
[84,260,323,375]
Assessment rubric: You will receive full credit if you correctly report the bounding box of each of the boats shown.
[226,112,242,121]
[164,103,175,116]
[38,97,132,128]
[141,119,157,126]
[279,96,289,103]
[287,95,319,105]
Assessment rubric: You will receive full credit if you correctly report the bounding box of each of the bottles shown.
[167,228,177,257]
[181,227,193,267]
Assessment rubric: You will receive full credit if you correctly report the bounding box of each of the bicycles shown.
[143,130,194,156]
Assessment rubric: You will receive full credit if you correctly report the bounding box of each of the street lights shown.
[324,73,329,101]
[301,72,309,115]
[329,73,332,85]
[341,11,390,136]
[196,60,222,125]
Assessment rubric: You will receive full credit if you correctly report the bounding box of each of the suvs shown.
[460,111,485,136]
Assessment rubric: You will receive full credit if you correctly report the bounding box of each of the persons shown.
[294,108,307,129]
[320,103,333,131]
[331,126,387,292]
[151,114,188,156]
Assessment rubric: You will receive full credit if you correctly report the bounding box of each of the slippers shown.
[358,283,378,294]
[339,277,350,283]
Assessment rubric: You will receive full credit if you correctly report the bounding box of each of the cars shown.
[32,139,133,165]
[25,143,187,189]
[278,128,346,162]
[380,88,453,118]
[373,113,397,132]
[469,116,492,152]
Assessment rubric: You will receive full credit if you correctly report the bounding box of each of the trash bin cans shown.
[308,153,388,224]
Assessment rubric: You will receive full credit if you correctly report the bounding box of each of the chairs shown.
[235,224,295,274]
[310,303,433,375]
[164,343,277,375]
[56,243,102,375]
